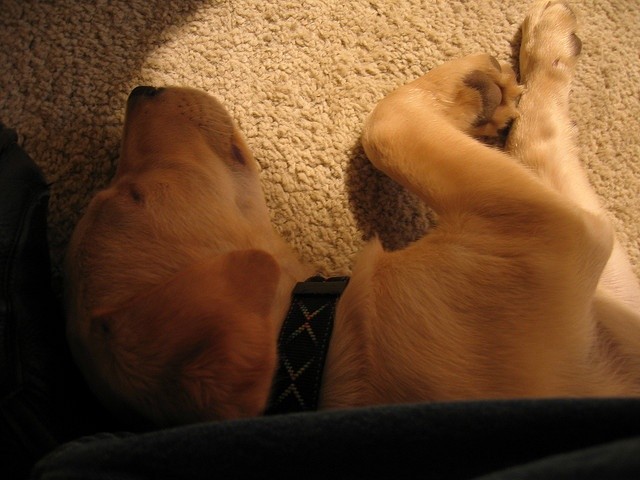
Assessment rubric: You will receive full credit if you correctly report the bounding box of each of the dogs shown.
[50,1,640,424]
